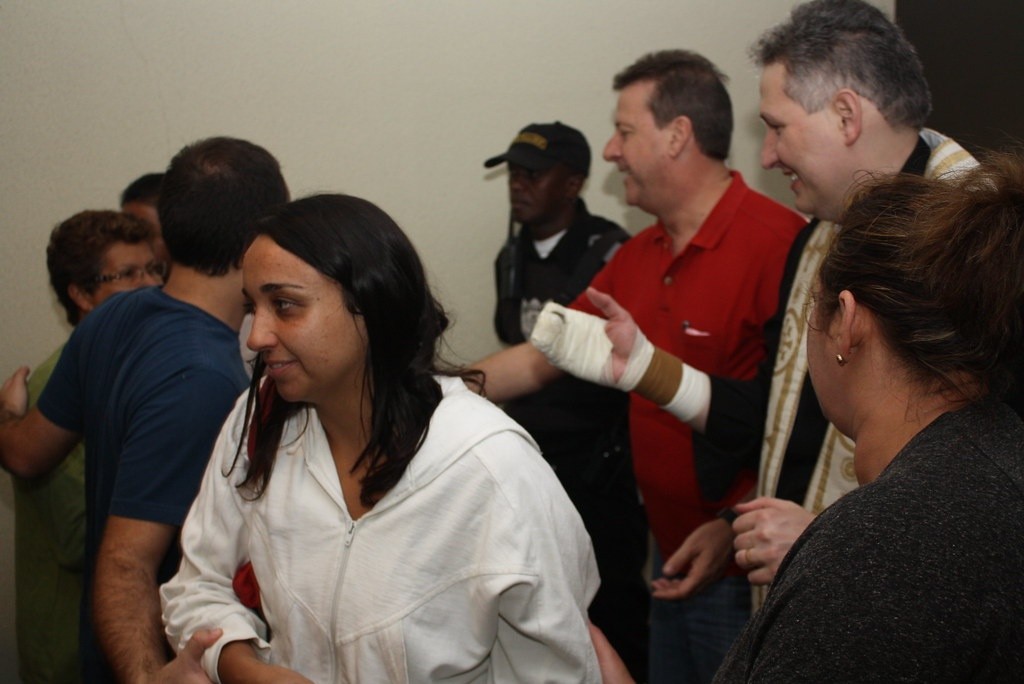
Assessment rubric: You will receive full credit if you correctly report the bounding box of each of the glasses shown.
[99,262,166,287]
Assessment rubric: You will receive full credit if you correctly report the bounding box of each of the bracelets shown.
[720,509,738,523]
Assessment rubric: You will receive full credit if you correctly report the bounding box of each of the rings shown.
[745,549,756,569]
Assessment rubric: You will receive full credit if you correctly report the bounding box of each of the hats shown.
[486,122,591,172]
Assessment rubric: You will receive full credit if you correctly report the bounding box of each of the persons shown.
[585,153,1023,684]
[120,171,175,275]
[480,118,650,683]
[157,193,607,683]
[0,136,291,684]
[456,44,810,682]
[527,0,995,615]
[11,206,163,684]
